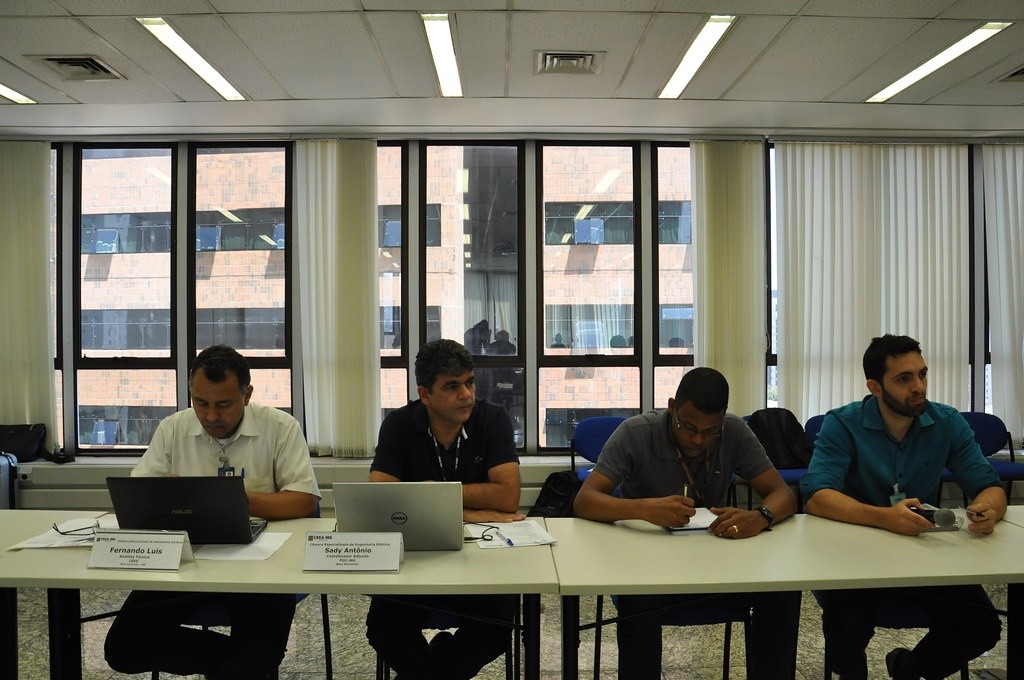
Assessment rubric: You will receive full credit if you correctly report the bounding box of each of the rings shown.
[733,525,738,533]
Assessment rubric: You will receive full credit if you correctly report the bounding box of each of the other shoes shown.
[886,648,919,680]
[839,652,868,680]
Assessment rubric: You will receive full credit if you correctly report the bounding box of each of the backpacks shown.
[747,408,814,469]
[525,471,583,518]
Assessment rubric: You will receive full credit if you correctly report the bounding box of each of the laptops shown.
[331,481,464,551]
[105,476,268,546]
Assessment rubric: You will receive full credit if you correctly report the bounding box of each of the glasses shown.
[674,401,724,437]
[52,520,99,536]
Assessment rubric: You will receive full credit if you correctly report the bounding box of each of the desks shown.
[0,508,1023,680]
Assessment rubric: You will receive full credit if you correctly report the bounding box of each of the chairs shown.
[146,407,1024,680]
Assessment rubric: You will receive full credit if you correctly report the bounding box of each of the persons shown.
[573,367,802,680]
[467,319,516,411]
[551,333,689,348]
[365,339,526,680]
[103,345,322,680]
[803,334,1008,680]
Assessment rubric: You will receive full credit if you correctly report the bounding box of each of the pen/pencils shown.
[241,468,244,479]
[684,483,688,497]
[960,506,985,518]
[496,532,513,545]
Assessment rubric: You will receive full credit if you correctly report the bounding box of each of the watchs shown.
[755,506,777,530]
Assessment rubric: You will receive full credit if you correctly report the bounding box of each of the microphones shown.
[909,507,956,527]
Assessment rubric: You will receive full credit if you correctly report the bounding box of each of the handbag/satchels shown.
[0,423,46,462]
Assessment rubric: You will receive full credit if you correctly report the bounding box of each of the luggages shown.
[0,452,18,509]
